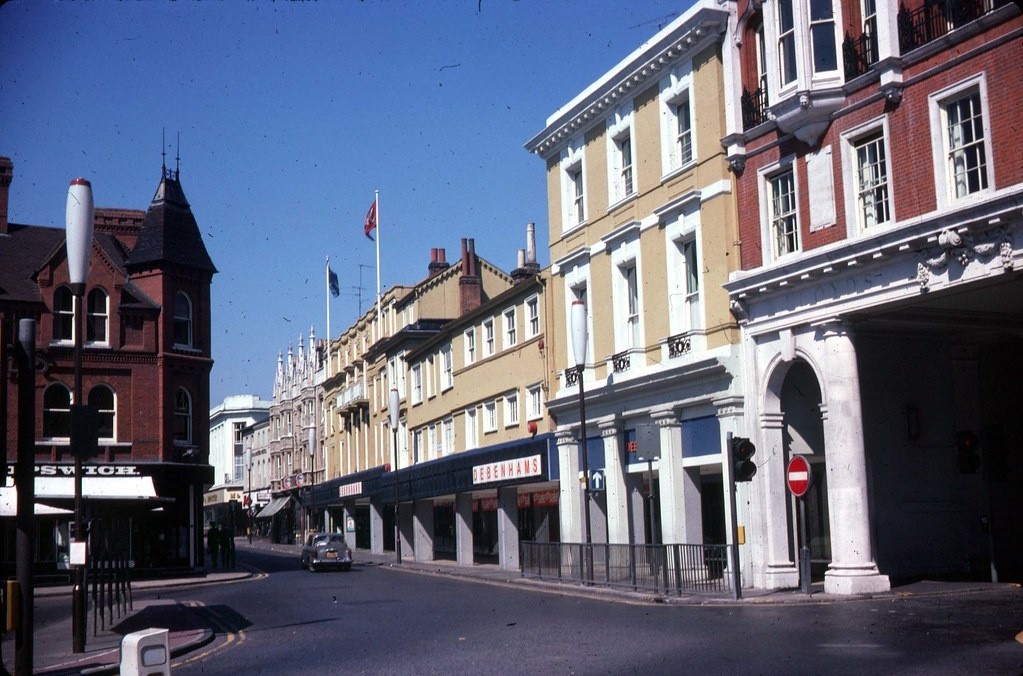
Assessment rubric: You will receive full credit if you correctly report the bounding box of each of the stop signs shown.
[786,455,812,497]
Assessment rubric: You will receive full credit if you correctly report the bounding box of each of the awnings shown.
[6,476,158,499]
[256,495,294,519]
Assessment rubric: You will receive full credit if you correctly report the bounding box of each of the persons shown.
[220,524,234,570]
[249,532,253,544]
[207,521,220,568]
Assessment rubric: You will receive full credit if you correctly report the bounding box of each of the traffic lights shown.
[731,436,759,483]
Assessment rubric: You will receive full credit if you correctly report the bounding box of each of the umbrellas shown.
[0,487,74,516]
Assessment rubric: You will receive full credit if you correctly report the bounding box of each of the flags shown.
[365,199,377,241]
[329,266,339,298]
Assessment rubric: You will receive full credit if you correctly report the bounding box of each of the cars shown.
[301,533,353,571]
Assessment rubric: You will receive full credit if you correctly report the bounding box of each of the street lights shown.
[570,299,596,586]
[308,422,319,537]
[61,176,98,653]
[389,387,407,566]
[244,445,258,543]
